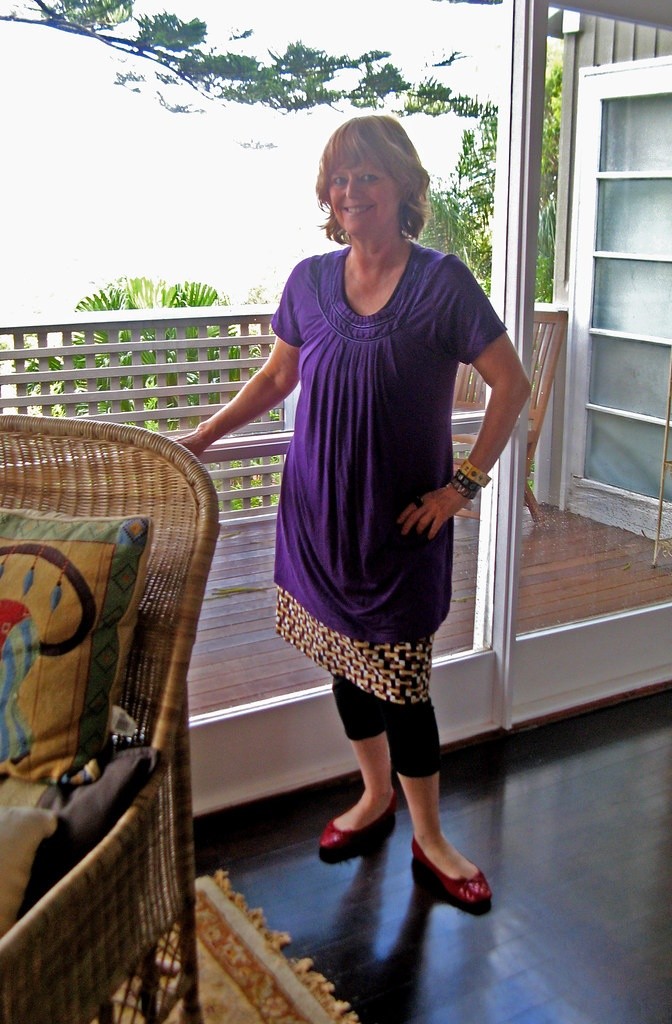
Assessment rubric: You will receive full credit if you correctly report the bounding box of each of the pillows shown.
[1,501,157,787]
[1,745,160,943]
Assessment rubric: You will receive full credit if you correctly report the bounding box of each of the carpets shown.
[87,867,364,1024]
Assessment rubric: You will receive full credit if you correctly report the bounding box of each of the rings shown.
[413,495,425,509]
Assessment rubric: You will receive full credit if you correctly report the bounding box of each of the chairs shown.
[445,303,568,525]
[0,414,221,1023]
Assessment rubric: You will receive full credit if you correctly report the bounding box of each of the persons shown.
[173,116,532,906]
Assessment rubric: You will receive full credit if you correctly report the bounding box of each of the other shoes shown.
[412,833,492,903]
[320,789,398,848]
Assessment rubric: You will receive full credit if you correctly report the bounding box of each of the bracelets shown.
[451,459,491,499]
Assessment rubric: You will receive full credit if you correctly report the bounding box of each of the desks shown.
[164,403,491,465]
